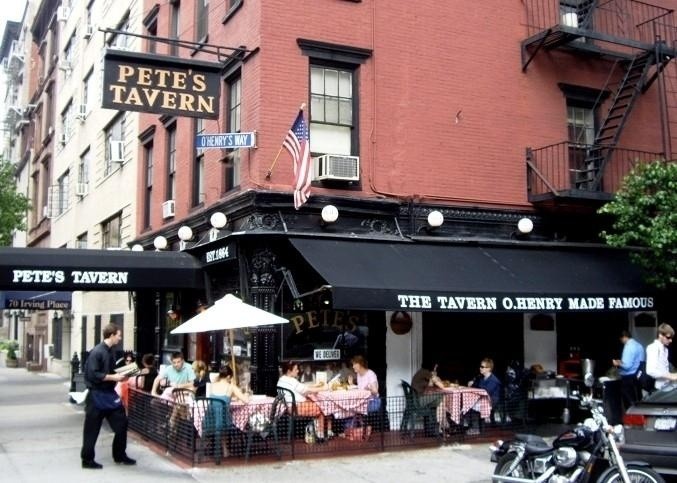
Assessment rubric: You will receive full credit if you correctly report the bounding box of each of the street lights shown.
[4,310,25,342]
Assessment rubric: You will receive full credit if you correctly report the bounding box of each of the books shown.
[114,362,149,376]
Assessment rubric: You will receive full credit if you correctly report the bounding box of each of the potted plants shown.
[0,339,21,368]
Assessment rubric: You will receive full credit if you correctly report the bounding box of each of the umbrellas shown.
[170,293,289,365]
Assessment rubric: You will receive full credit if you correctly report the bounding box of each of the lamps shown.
[52,309,58,318]
[178,223,200,242]
[416,208,444,234]
[18,308,25,317]
[509,216,533,240]
[315,204,341,228]
[196,298,210,313]
[208,209,236,232]
[152,234,172,250]
[166,295,181,320]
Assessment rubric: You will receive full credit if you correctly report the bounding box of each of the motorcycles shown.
[487,373,666,483]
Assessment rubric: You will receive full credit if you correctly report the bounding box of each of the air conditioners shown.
[159,200,175,218]
[107,140,124,163]
[81,24,93,37]
[73,101,87,117]
[311,153,361,182]
[56,4,70,20]
[74,182,88,197]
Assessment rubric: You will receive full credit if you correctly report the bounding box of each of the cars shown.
[617,376,677,482]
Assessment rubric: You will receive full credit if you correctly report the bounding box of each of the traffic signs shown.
[194,130,255,148]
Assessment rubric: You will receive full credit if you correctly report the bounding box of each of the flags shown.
[283,108,312,211]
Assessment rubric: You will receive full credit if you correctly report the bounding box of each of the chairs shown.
[119,368,649,466]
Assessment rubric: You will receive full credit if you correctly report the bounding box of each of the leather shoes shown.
[81,458,104,469]
[113,454,137,465]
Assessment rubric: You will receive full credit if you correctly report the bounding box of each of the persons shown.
[169,360,211,439]
[212,366,249,458]
[127,354,161,395]
[150,352,197,431]
[81,323,137,468]
[612,329,645,403]
[115,352,145,402]
[645,323,677,390]
[465,358,501,436]
[276,355,381,443]
[412,357,451,438]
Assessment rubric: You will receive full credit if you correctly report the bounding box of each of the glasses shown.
[480,365,492,370]
